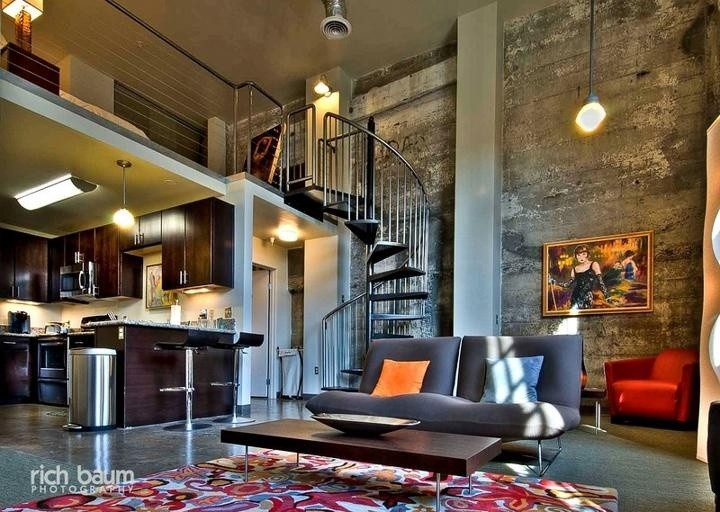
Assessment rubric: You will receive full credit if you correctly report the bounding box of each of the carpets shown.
[1,448,616,511]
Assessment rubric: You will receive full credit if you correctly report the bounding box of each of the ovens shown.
[38,335,67,406]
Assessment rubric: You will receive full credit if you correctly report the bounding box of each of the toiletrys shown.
[170,298,181,325]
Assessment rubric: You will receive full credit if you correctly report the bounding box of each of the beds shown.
[220,417,500,511]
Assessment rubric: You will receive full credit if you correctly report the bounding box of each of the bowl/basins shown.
[311,412,423,437]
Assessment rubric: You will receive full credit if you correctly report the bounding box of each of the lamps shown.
[112,159,134,232]
[573,2,607,135]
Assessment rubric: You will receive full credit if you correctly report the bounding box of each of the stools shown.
[210,330,266,424]
[151,328,218,433]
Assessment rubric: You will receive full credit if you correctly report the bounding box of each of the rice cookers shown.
[7,311,31,334]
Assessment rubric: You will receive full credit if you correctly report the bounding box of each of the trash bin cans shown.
[63,348,117,433]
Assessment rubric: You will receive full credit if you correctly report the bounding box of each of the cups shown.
[170,305,181,325]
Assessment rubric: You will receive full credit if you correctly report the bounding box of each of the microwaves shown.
[59,261,96,303]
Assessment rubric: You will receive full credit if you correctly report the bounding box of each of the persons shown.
[547,244,611,309]
[622,250,638,279]
[613,261,621,268]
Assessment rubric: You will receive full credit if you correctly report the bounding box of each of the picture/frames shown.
[144,264,177,310]
[538,228,656,315]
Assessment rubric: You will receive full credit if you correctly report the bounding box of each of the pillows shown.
[371,356,429,396]
[482,354,544,409]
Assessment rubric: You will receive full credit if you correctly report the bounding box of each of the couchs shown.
[603,346,697,427]
[303,334,585,477]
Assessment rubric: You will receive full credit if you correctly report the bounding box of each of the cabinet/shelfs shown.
[161,196,235,294]
[120,210,162,257]
[65,228,94,266]
[48,236,65,303]
[0,226,48,301]
[94,225,143,300]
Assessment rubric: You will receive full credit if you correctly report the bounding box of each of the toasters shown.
[44,321,66,335]
